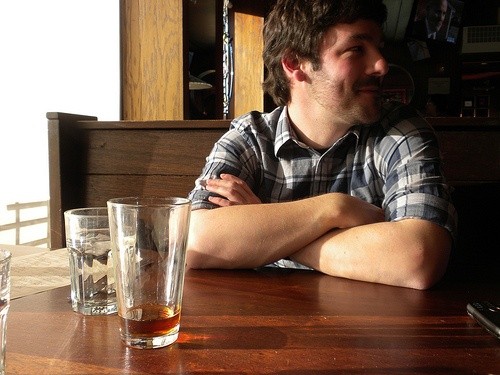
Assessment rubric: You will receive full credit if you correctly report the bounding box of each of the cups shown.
[0,249,12,375]
[64,207,120,315]
[106,196,192,350]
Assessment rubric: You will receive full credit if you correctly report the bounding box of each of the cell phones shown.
[467,302,500,340]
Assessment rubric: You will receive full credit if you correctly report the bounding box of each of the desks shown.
[0,244,500,375]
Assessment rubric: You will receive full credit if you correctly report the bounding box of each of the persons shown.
[167,0,458,290]
[411,0,448,41]
